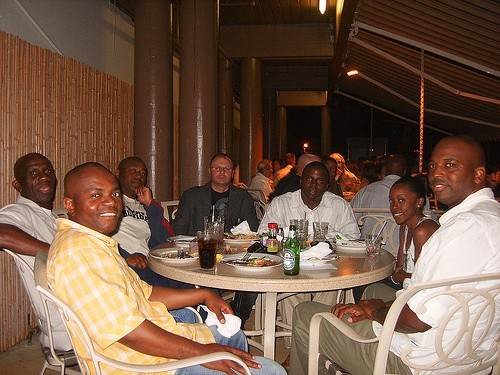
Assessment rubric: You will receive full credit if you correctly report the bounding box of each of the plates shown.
[148,247,198,264]
[333,245,367,254]
[221,252,285,272]
[167,236,197,248]
[223,239,261,248]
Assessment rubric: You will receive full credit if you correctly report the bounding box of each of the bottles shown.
[283,224,300,276]
[266,223,279,255]
[276,228,284,252]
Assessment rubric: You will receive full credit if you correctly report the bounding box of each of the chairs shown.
[307,273,500,375]
[353,207,431,261]
[35,283,251,375]
[1,248,83,375]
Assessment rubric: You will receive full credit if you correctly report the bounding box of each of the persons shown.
[0,153,197,354]
[116,135,500,375]
[46,163,288,375]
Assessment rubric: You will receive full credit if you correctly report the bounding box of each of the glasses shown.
[211,167,232,172]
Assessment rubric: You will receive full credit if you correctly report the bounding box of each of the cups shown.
[289,219,309,252]
[198,230,219,271]
[312,222,329,242]
[203,215,224,253]
[365,234,382,257]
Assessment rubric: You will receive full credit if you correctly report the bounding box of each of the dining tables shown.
[147,233,397,361]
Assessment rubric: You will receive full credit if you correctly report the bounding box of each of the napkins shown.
[297,242,339,271]
[168,235,196,242]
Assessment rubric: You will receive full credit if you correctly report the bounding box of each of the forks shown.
[219,253,251,265]
